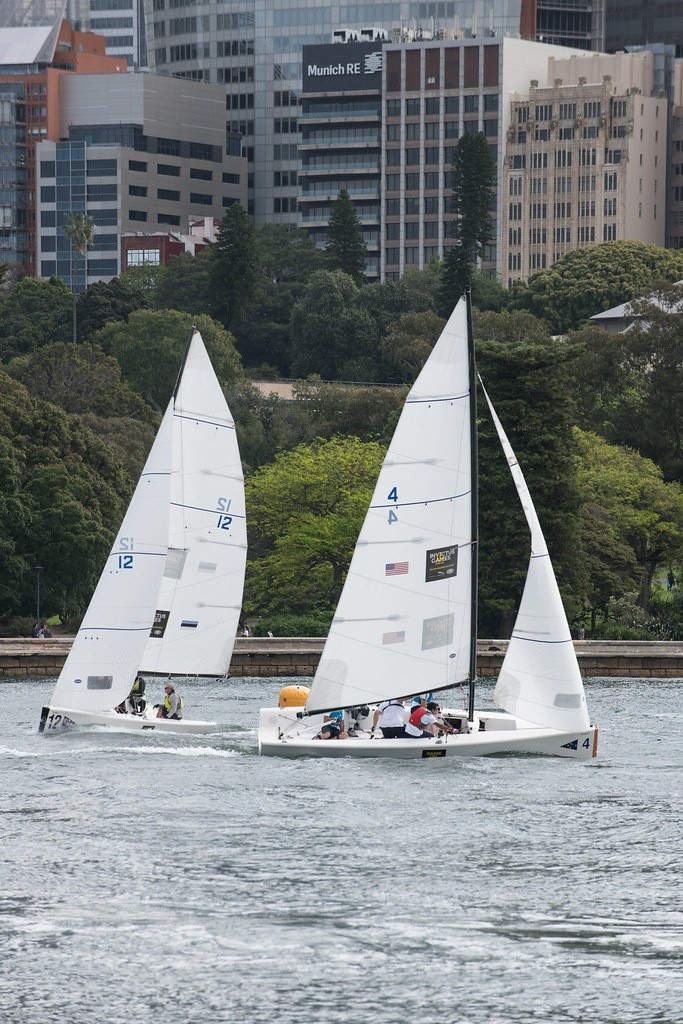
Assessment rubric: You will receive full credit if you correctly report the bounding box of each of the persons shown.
[312,692,459,739]
[241,623,274,637]
[153,684,184,720]
[31,623,53,638]
[127,676,146,717]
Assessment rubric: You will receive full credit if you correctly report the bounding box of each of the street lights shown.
[32,565,44,638]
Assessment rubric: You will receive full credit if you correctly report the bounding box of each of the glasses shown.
[165,687,170,689]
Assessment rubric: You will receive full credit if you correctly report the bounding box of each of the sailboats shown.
[33,313,257,736]
[255,272,601,761]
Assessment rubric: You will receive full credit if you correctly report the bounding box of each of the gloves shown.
[153,704,159,709]
[163,716,168,719]
